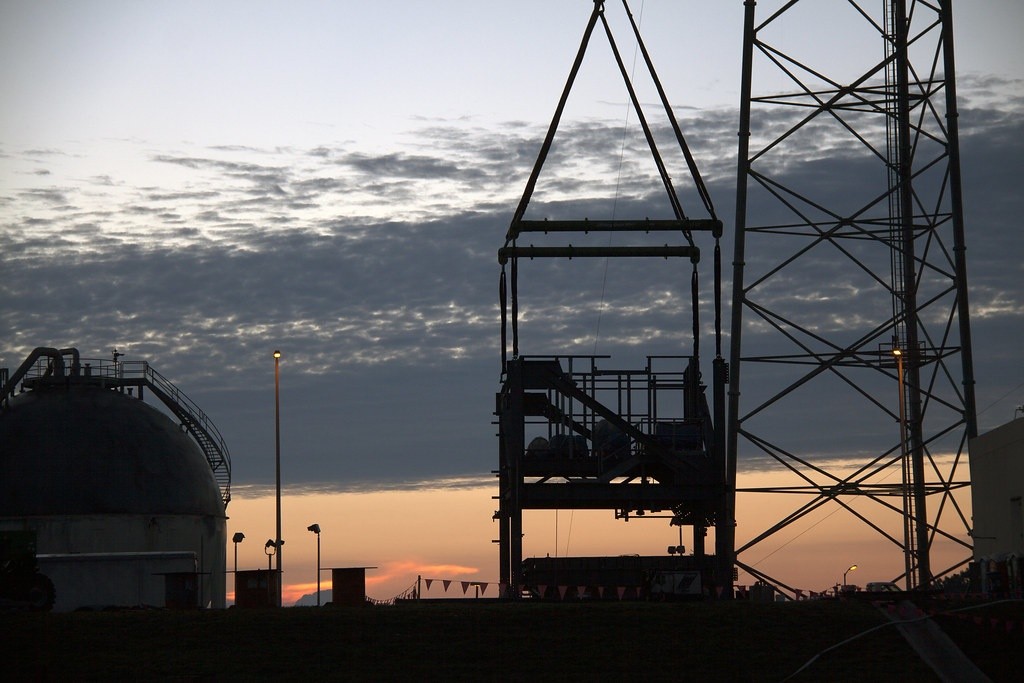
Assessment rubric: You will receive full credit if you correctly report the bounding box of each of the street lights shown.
[265,540,277,569]
[308,524,321,606]
[231,532,245,572]
[844,565,857,585]
[274,349,283,605]
[891,345,914,593]
